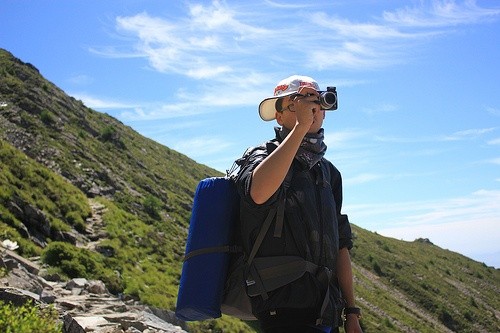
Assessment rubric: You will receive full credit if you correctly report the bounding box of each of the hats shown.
[259,75,321,122]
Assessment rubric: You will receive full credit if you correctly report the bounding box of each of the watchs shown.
[344,307,362,320]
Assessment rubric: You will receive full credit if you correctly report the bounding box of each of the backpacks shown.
[174,143,339,321]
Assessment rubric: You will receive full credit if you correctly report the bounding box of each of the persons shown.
[230,73,364,333]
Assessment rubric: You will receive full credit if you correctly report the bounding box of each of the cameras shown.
[310,86,337,110]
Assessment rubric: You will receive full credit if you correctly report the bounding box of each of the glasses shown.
[276,103,296,113]
